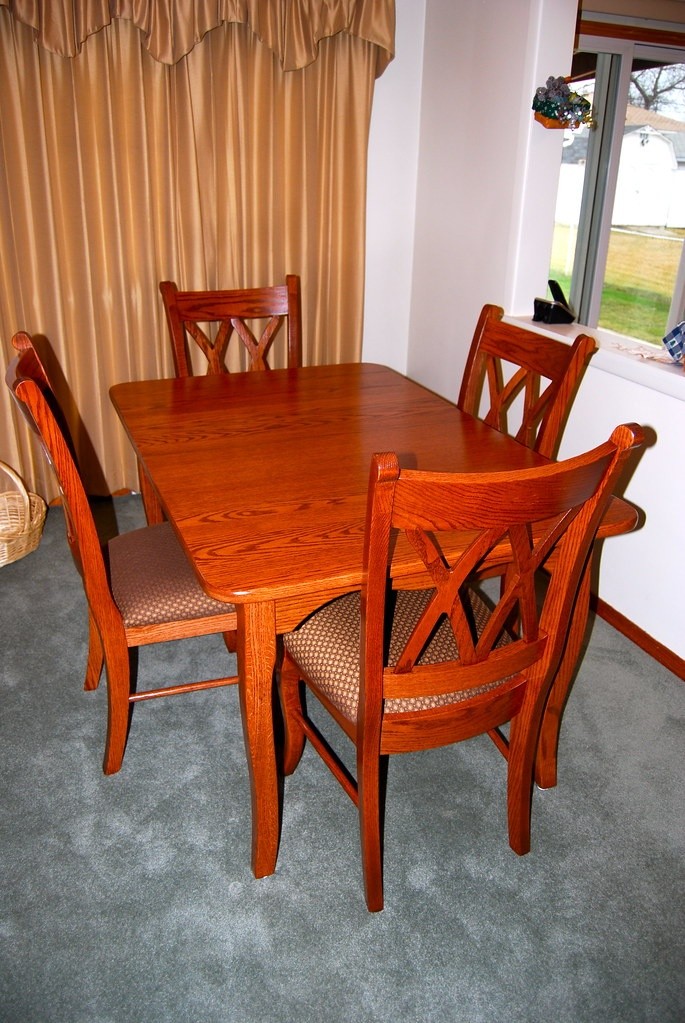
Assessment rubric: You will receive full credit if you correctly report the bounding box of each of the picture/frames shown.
[531,93,591,130]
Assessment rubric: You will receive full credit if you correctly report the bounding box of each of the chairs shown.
[458,303,596,638]
[157,273,302,378]
[280,424,644,913]
[3,330,238,774]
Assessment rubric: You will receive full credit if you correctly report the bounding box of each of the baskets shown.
[0,461,46,569]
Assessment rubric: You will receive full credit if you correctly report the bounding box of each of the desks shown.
[109,363,638,879]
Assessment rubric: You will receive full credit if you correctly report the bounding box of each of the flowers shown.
[536,75,597,129]
